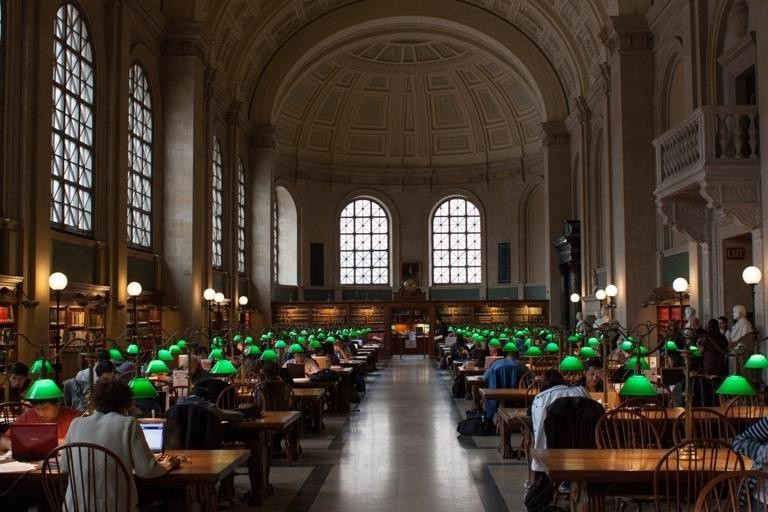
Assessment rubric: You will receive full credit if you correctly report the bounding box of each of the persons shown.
[592,305,611,328]
[684,306,703,329]
[407,264,416,279]
[729,304,753,349]
[575,311,587,332]
[1,333,359,512]
[432,316,767,511]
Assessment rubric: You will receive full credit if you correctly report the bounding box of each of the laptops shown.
[132,418,166,475]
[287,364,304,377]
[10,423,58,460]
[445,337,457,345]
[485,357,504,368]
